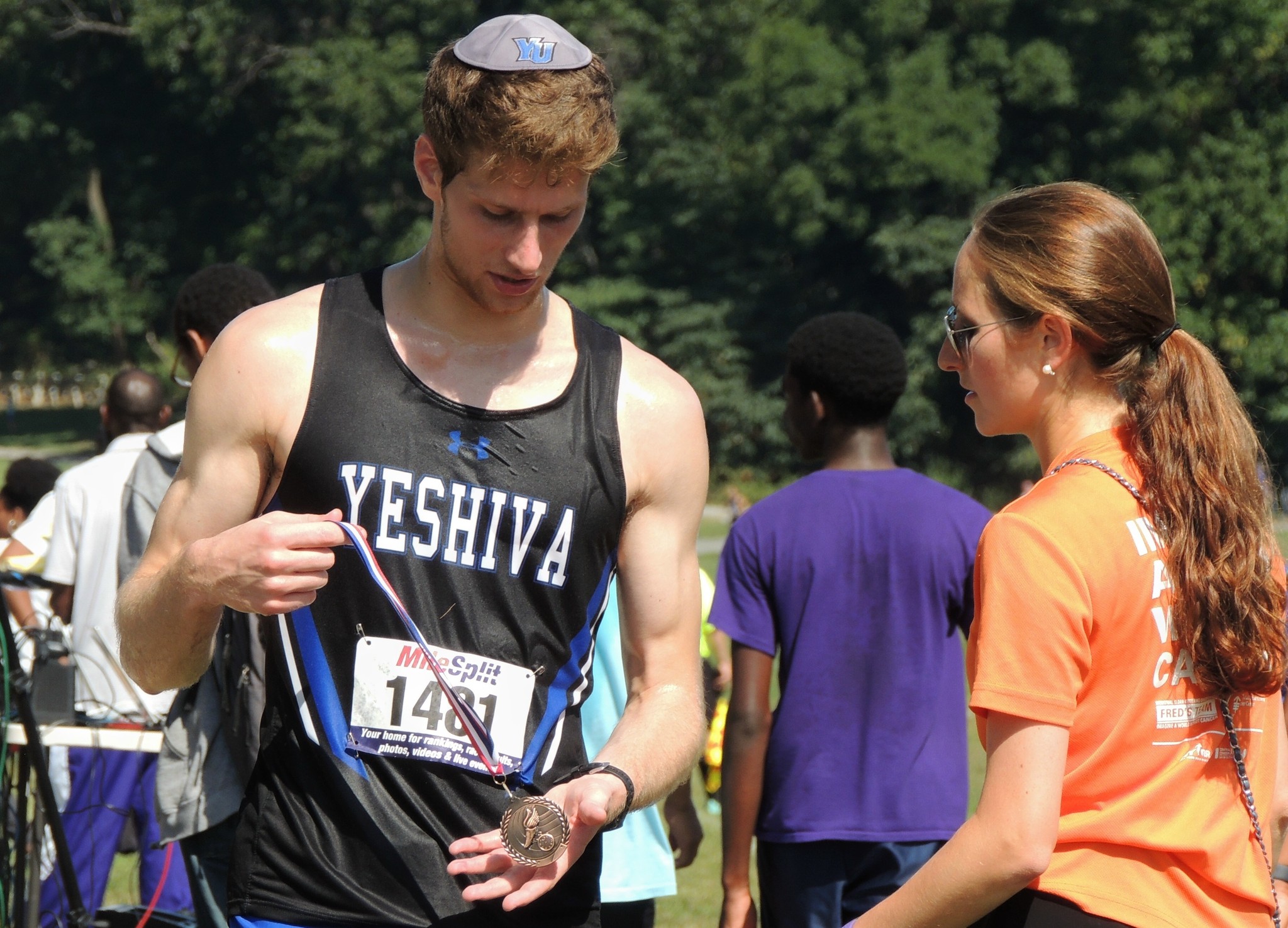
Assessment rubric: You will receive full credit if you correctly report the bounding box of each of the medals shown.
[499,794,571,868]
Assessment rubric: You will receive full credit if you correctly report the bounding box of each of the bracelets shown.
[553,762,635,842]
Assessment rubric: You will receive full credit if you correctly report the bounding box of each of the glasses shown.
[170,336,192,388]
[943,306,1042,357]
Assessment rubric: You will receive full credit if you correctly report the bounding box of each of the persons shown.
[114,12,710,928]
[705,308,996,928]
[578,560,731,928]
[828,180,1288,928]
[0,261,286,928]
